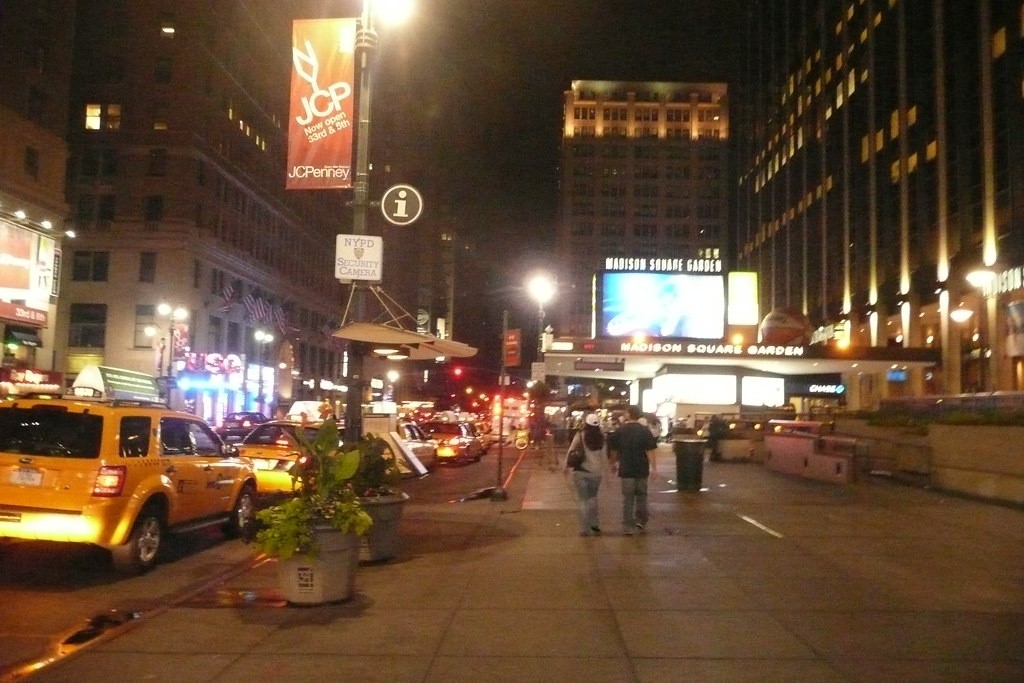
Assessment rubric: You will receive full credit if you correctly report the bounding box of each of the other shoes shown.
[580,526,601,536]
[621,522,649,536]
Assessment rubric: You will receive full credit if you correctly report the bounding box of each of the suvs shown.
[0,387,258,575]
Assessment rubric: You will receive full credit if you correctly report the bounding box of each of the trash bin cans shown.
[670,439,708,491]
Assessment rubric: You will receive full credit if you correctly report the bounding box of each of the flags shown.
[217,284,343,348]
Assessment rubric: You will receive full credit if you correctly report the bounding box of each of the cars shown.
[395,404,491,469]
[222,420,376,500]
[216,411,274,440]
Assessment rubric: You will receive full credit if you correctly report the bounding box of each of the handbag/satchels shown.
[566,431,586,467]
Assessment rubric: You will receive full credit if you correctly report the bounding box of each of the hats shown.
[585,413,600,426]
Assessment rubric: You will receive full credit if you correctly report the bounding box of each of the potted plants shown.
[255,418,374,608]
[336,431,411,562]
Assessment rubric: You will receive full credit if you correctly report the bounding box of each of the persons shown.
[530,409,719,448]
[610,406,657,535]
[1005,325,1024,390]
[565,414,611,537]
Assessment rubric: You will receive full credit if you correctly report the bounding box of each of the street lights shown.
[529,277,553,361]
[340,1,416,564]
